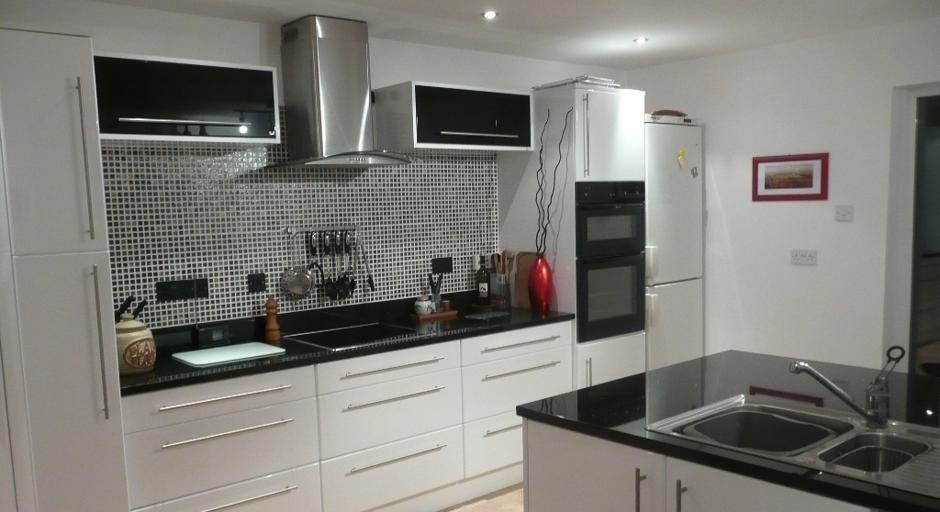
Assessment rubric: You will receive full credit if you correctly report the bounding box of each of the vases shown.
[528,255,556,317]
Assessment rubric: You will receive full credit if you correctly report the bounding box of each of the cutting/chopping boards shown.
[513,251,541,311]
[171,340,286,368]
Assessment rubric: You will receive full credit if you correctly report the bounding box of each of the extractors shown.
[268,12,412,172]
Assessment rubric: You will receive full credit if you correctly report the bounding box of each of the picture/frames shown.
[751,151,829,202]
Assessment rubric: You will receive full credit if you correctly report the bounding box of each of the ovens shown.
[575,179,645,342]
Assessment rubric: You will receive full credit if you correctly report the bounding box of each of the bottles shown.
[476,256,490,305]
[264,296,281,341]
[414,294,436,315]
[114,313,157,376]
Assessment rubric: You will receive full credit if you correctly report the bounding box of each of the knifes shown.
[131,298,149,319]
[115,296,134,321]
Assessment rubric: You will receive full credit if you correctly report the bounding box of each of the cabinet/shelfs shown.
[315,335,465,512]
[496,87,645,186]
[1,22,112,257]
[372,81,534,157]
[1,247,131,511]
[461,315,573,508]
[93,48,281,147]
[575,332,647,392]
[121,365,323,511]
[522,417,868,512]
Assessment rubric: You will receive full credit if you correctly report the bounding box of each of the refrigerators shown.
[643,112,704,372]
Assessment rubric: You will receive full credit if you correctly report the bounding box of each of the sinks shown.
[670,404,854,458]
[818,433,930,474]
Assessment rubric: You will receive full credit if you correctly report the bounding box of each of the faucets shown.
[789,346,905,427]
